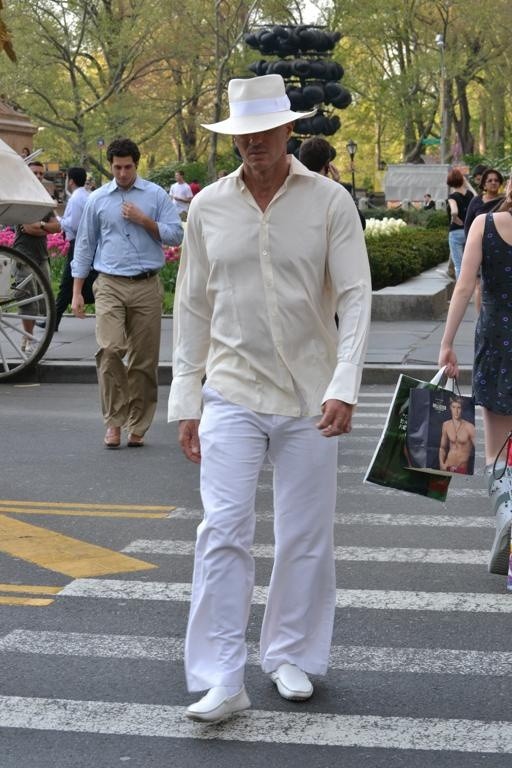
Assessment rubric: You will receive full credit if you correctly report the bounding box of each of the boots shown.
[486,458,512,576]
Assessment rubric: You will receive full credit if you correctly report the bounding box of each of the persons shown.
[71,139,184,448]
[21,147,31,159]
[439,394,475,474]
[170,170,193,216]
[11,161,62,355]
[189,179,201,196]
[437,179,512,575]
[34,166,91,331]
[166,74,373,723]
[298,138,366,236]
[423,164,507,315]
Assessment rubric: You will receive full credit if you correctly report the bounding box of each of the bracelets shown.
[39,220,45,229]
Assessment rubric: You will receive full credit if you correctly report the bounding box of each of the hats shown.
[199,72,320,138]
[298,138,337,172]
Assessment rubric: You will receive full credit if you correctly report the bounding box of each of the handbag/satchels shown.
[402,367,476,478]
[363,364,457,503]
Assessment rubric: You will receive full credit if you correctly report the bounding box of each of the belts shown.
[101,268,162,280]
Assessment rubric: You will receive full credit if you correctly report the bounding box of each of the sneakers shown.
[20,337,52,355]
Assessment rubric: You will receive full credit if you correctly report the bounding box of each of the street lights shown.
[379,160,387,169]
[435,33,452,163]
[346,137,359,202]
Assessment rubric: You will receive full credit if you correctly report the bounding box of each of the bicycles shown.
[0,245,58,377]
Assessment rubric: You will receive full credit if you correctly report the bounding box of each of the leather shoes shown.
[267,659,316,704]
[104,425,123,448]
[183,684,252,723]
[34,318,59,334]
[127,430,144,449]
